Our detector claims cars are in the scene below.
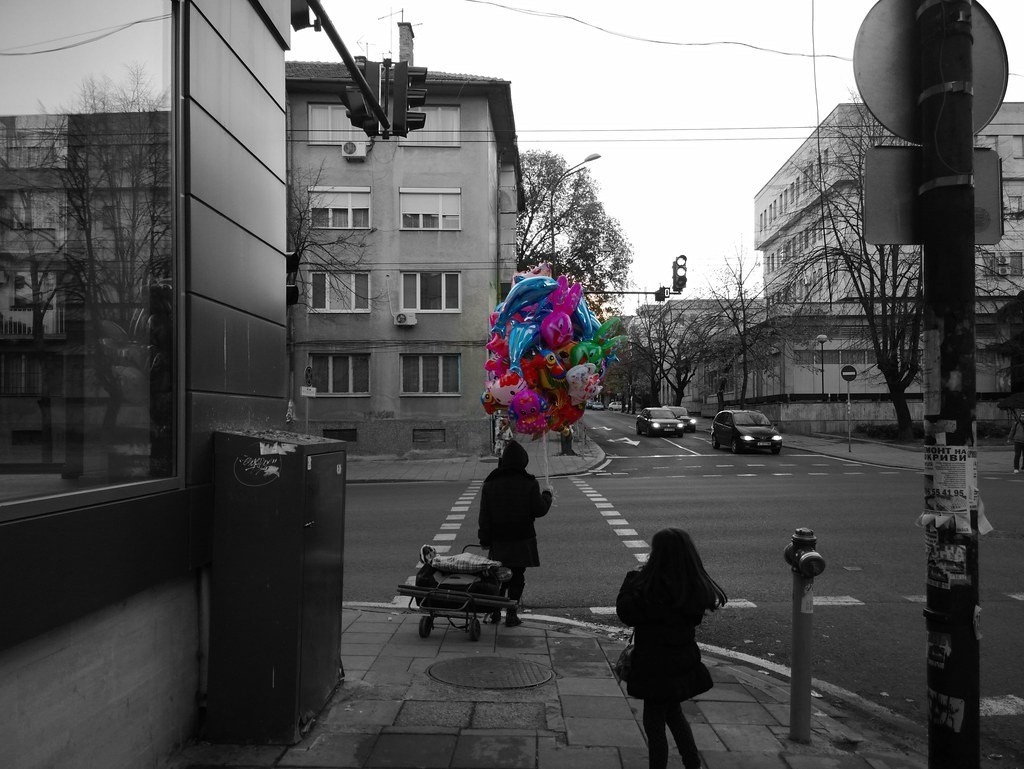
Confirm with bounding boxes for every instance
[586,401,605,410]
[664,406,696,432]
[711,410,783,454]
[608,402,629,411]
[637,407,685,439]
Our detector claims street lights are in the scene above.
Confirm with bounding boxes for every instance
[817,335,828,396]
[549,153,602,280]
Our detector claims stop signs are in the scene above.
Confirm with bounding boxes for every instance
[841,365,857,381]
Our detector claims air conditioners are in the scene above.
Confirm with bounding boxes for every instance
[997,256,1010,265]
[394,312,415,326]
[342,142,366,159]
[998,267,1011,275]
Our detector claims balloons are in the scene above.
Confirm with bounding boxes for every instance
[481,261,630,439]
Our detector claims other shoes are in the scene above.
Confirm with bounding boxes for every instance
[1014,467,1024,473]
[491,611,502,624]
[506,612,523,627]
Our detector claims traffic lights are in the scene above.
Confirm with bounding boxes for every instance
[672,255,687,291]
[394,60,429,138]
[338,59,381,136]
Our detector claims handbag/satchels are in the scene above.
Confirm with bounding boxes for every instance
[615,645,634,681]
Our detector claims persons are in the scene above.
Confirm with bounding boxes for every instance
[478,441,554,627]
[1006,412,1024,473]
[616,527,728,769]
[494,419,513,469]
